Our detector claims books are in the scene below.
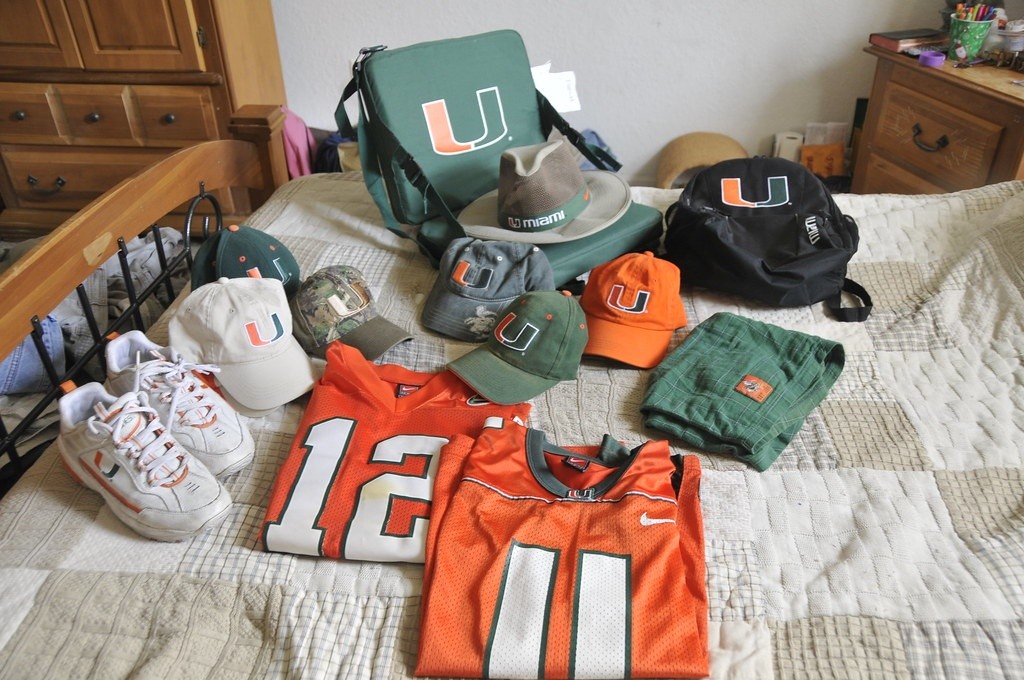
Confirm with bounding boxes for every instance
[869,27,951,54]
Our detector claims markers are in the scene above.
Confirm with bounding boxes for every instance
[956,2,997,21]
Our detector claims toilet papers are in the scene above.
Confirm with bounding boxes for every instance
[773,131,804,169]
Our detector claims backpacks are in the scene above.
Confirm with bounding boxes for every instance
[663,154,874,320]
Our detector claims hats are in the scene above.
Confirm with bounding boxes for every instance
[418,135,688,409]
[166,224,413,413]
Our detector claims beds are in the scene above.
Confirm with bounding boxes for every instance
[0,102,1024,679]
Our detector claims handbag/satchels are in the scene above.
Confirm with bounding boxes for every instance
[352,29,550,227]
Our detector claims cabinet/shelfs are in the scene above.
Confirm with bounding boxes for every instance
[846,31,1024,193]
[0,1,293,242]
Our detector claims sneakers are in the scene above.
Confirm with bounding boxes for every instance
[54,330,255,541]
